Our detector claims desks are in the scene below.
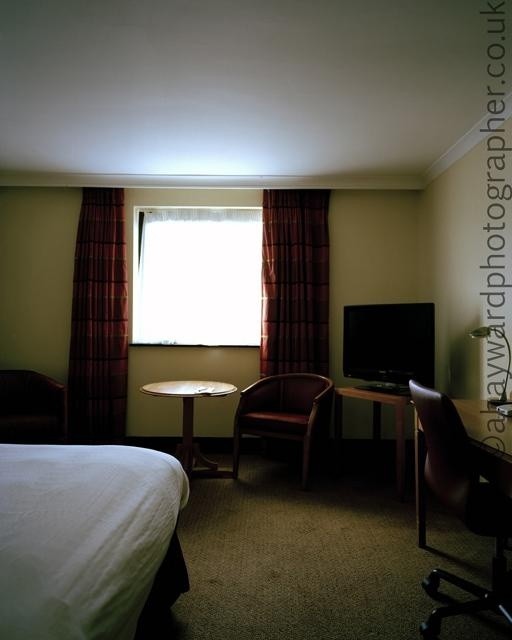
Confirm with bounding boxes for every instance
[333,386,411,506]
[410,399,512,547]
[138,379,238,478]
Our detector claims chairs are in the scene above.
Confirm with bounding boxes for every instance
[232,372,335,492]
[0,371,68,445]
[408,379,512,640]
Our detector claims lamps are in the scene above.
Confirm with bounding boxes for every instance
[469,327,512,406]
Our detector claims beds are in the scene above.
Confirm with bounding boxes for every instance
[1,445,189,640]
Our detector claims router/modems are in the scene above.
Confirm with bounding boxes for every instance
[496,403,512,416]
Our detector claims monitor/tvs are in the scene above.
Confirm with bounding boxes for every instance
[343,302,435,395]
